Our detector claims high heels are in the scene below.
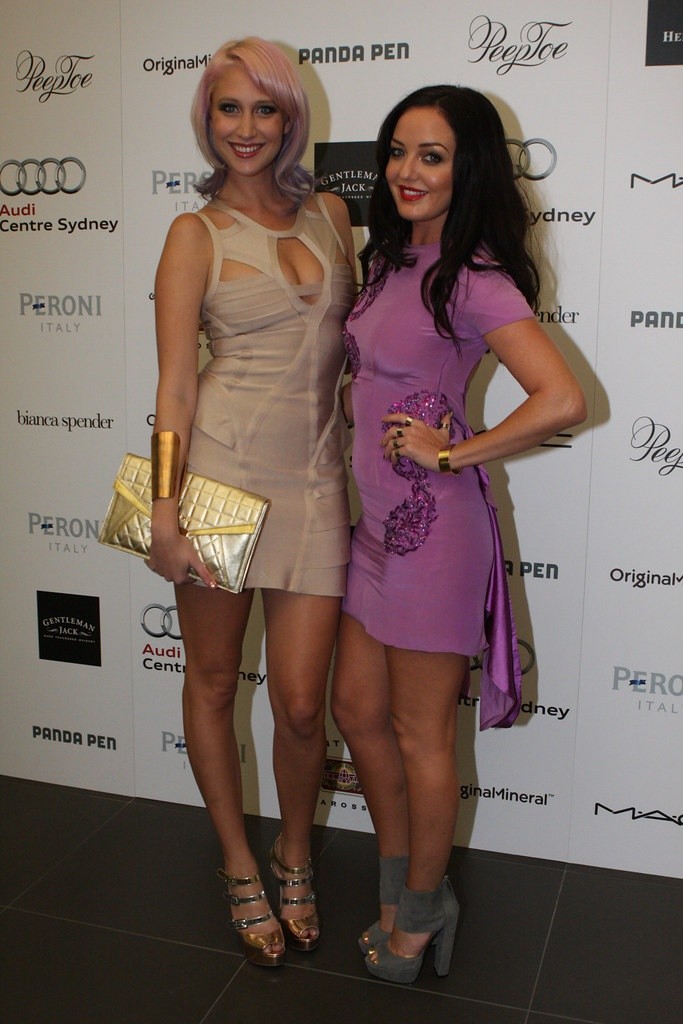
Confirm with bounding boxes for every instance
[268,838,320,952]
[366,875,461,982]
[218,859,286,968]
[356,855,416,956]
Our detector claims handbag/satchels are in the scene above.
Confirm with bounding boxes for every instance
[98,452,270,595]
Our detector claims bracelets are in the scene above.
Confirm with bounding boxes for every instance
[438,444,463,477]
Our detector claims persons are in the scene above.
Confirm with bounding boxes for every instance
[149,37,357,968]
[329,84,587,984]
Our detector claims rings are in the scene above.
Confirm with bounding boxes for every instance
[395,448,400,457]
[439,423,450,430]
[392,437,399,448]
[405,415,413,426]
[397,427,403,437]
